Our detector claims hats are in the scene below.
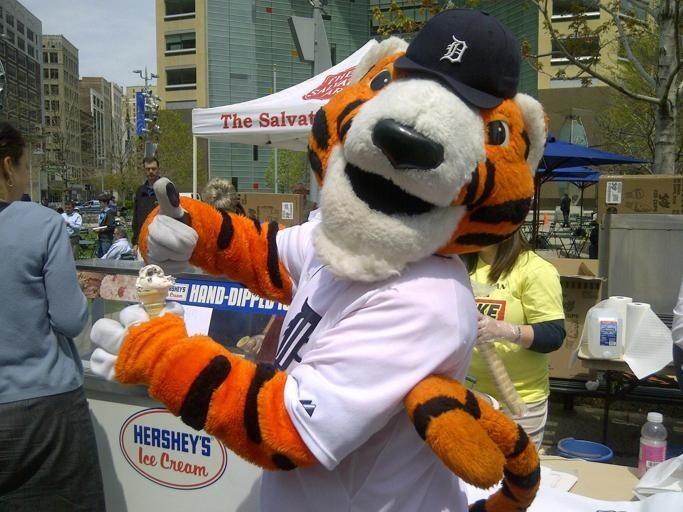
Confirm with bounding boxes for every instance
[98,208,125,226]
[74,200,102,214]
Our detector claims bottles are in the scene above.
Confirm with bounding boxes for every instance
[393,9,521,109]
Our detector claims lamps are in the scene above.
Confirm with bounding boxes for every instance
[121,251,135,260]
[79,230,95,258]
[523,216,592,259]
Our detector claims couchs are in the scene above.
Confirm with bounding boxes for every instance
[84,184,88,191]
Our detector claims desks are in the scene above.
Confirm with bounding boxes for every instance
[132,66,163,158]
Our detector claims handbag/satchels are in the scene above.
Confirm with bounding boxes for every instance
[512,325,523,341]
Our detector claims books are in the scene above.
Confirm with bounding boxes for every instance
[547,260,606,382]
[239,193,304,228]
[597,176,683,214]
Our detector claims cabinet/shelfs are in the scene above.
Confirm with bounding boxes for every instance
[637,411,668,482]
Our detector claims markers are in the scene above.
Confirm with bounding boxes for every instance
[135,265,177,319]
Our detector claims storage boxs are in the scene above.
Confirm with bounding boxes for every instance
[543,314,682,445]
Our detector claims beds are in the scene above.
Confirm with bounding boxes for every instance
[528,133,655,258]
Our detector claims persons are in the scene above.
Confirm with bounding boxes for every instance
[669,281,683,389]
[463,229,568,455]
[201,177,244,217]
[0,124,106,509]
[92,195,131,261]
[293,182,319,224]
[59,200,82,260]
[131,156,164,259]
